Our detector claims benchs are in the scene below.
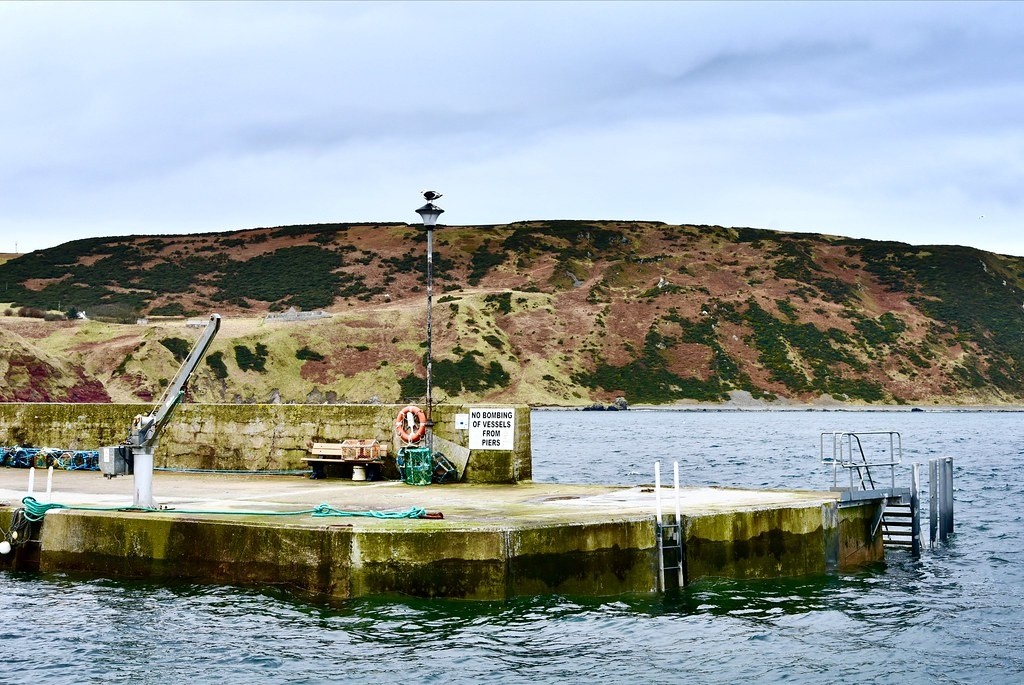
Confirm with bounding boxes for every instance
[186,320,210,328]
[300,442,387,480]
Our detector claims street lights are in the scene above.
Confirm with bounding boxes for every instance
[416,189,446,461]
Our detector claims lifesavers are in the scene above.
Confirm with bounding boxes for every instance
[396,406,426,442]
